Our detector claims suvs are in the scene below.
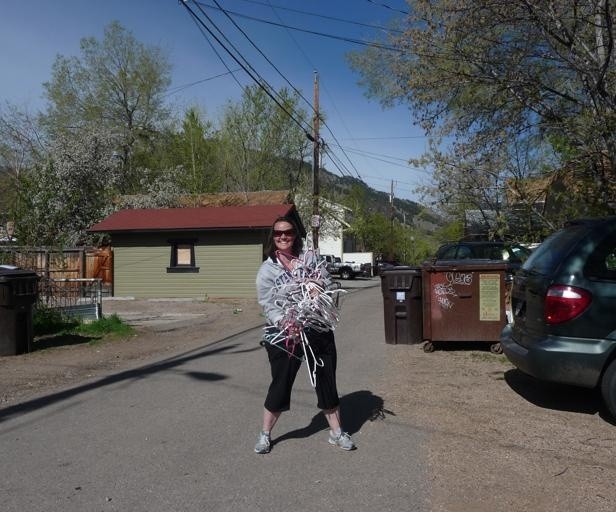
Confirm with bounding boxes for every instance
[500,215,616,418]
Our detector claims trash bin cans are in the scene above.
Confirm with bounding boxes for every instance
[0,265,41,357]
[377,259,511,354]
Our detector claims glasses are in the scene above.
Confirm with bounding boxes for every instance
[272,228,295,237]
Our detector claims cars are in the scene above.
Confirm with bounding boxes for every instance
[435,240,542,270]
[320,255,401,279]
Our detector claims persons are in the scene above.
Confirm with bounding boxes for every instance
[253,215,355,454]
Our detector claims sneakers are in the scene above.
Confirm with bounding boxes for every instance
[254,431,271,453]
[328,430,355,451]
